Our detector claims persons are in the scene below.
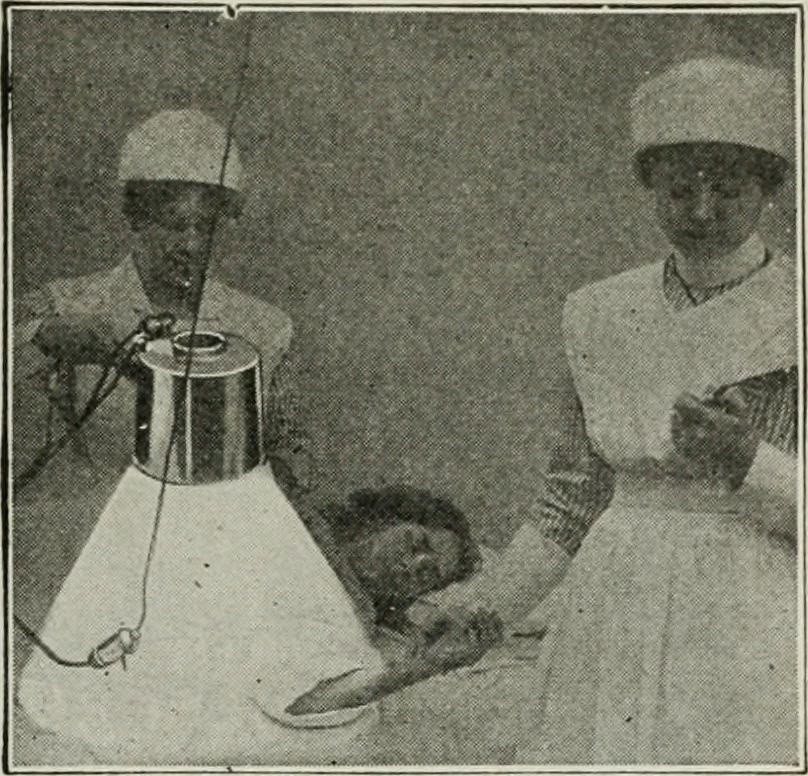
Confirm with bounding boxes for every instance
[12,108,293,678]
[286,483,505,718]
[398,53,797,766]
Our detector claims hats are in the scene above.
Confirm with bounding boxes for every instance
[627,54,795,190]
[118,108,242,195]
